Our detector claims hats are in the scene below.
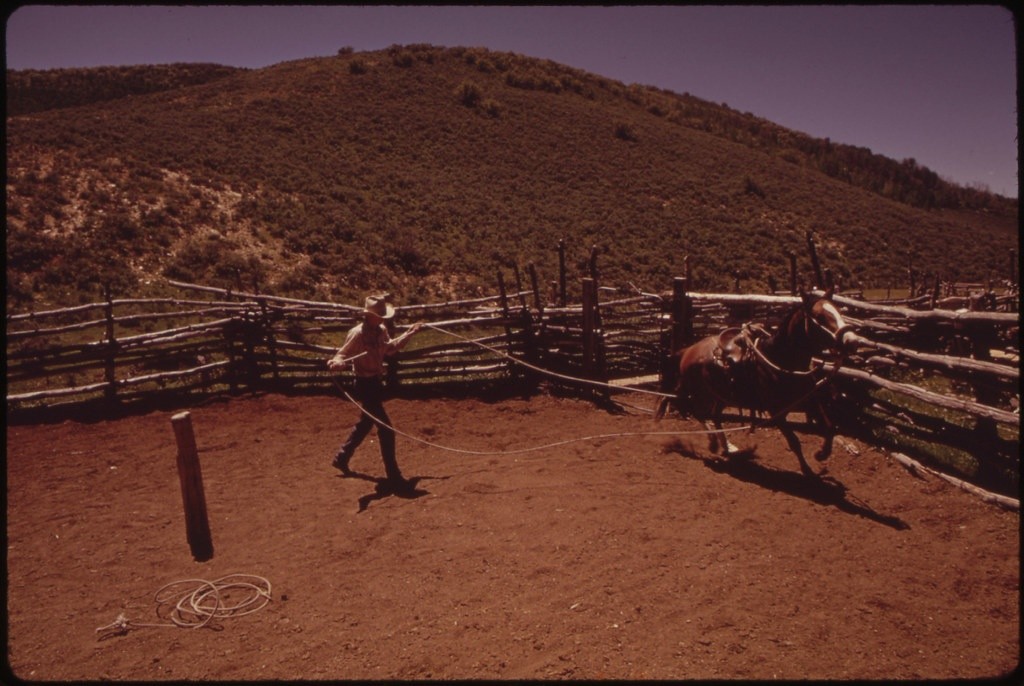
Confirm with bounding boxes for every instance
[358,295,395,319]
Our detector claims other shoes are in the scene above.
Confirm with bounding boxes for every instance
[389,477,410,487]
[332,459,357,476]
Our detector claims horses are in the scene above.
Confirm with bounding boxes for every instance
[655,284,858,486]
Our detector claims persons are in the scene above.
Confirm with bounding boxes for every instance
[326,294,422,492]
